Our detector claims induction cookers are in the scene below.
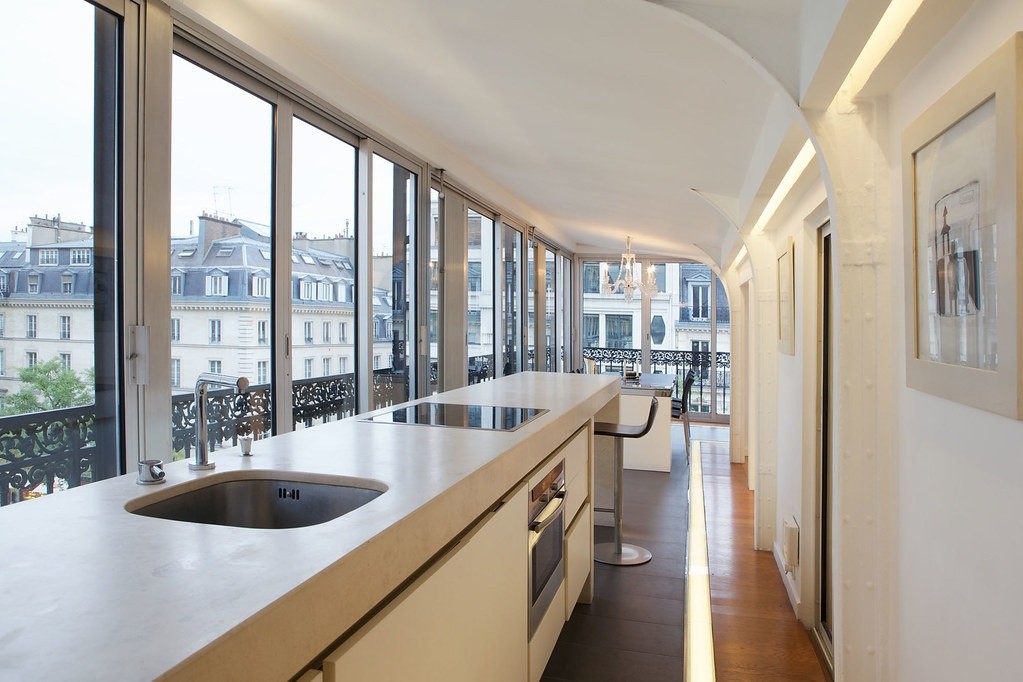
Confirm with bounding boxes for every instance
[355,401,550,433]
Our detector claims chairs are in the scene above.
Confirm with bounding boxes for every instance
[670,370,695,464]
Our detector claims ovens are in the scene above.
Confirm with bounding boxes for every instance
[527,459,568,642]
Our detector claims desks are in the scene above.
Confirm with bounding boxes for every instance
[601,371,677,397]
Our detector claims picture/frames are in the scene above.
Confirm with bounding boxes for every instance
[777,238,794,353]
[900,31,1023,423]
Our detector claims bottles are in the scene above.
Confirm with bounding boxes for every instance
[584,356,595,374]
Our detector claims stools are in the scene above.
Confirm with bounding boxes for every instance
[593,397,658,566]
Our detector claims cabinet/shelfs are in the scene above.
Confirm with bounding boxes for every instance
[297,418,595,682]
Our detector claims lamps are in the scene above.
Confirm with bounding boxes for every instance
[600,236,658,304]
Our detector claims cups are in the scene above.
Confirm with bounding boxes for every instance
[239,437,252,455]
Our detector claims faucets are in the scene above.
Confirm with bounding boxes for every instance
[188,372,249,471]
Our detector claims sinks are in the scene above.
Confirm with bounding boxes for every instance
[130,480,384,529]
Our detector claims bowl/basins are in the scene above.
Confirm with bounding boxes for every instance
[619,371,642,379]
